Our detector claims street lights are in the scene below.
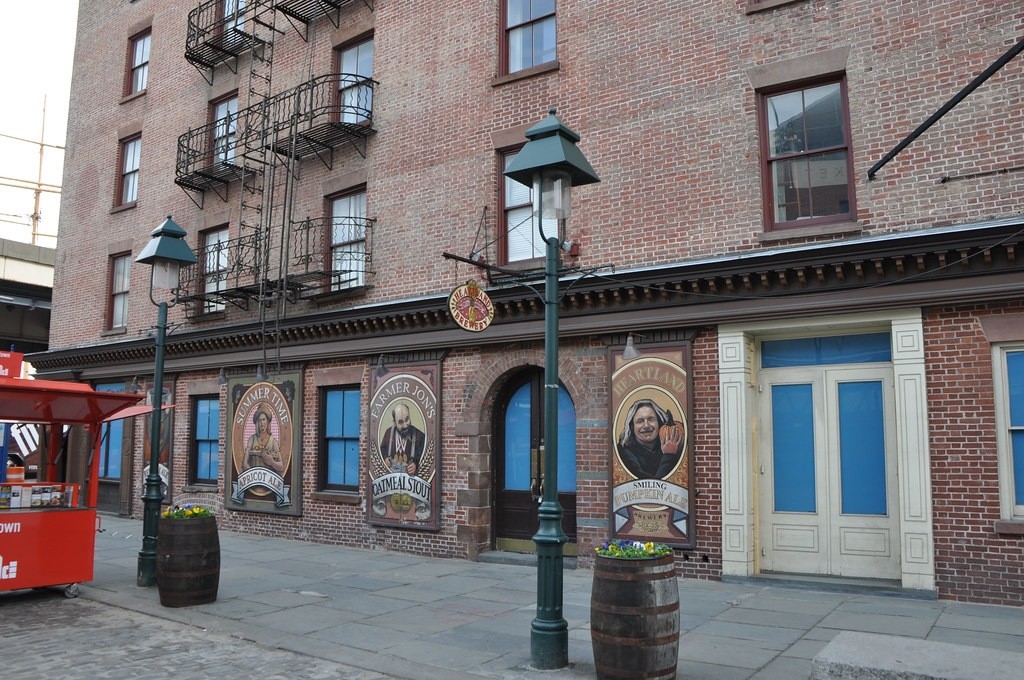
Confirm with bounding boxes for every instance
[501,108,601,665]
[133,213,199,587]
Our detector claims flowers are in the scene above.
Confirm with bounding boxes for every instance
[592,539,673,558]
[160,504,214,519]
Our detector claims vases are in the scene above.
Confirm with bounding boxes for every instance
[590,554,681,680]
[157,516,220,608]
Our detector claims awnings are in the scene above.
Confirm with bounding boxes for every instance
[0,293,51,310]
[0,382,176,466]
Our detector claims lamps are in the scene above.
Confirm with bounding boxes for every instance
[562,240,579,257]
[623,332,648,361]
[256,365,284,382]
[373,354,404,375]
[471,251,486,264]
[217,366,246,385]
[130,376,154,392]
